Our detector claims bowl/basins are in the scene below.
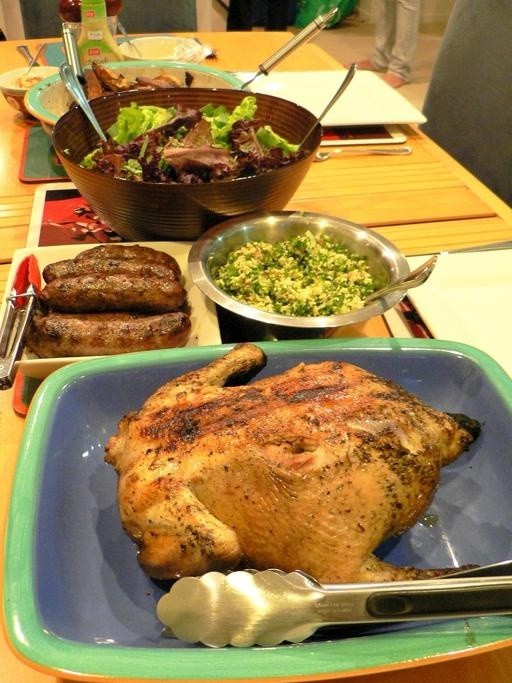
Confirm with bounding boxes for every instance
[188,211,412,327]
[0,66,64,126]
[23,60,254,141]
[118,36,206,63]
[50,87,324,243]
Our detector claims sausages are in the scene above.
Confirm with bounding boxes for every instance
[29,245,192,360]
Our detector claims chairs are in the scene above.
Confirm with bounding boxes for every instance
[18,0,198,40]
[417,0,512,207]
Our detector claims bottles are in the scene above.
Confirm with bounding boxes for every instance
[75,1,124,75]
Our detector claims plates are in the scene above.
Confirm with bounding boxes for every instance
[2,242,224,378]
[234,68,428,127]
[0,339,510,682]
[381,248,510,375]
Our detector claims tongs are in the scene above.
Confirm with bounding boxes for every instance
[0,254,38,391]
[156,558,511,648]
